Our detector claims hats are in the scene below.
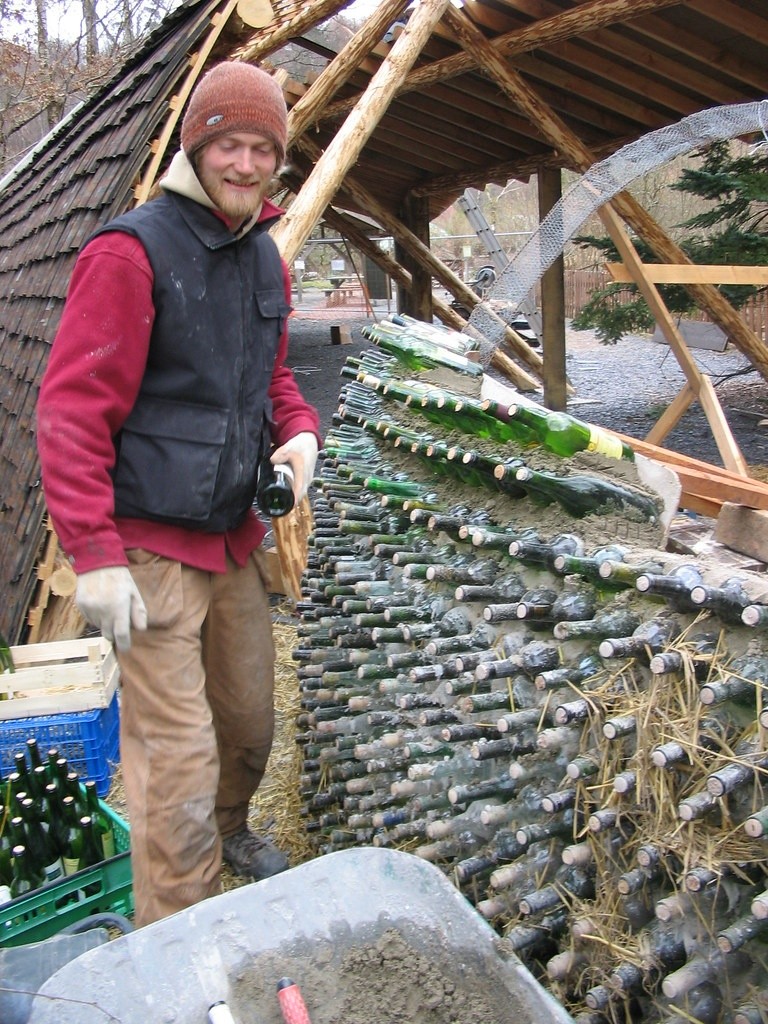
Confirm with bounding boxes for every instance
[181,61,287,176]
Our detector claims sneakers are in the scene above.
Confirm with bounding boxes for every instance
[222,826,288,879]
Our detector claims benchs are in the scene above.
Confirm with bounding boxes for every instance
[321,283,364,303]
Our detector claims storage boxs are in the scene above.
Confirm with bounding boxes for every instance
[0,691,136,949]
[331,324,354,346]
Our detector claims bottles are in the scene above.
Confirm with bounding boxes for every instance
[0,739,115,901]
[0,633,17,701]
[259,312,768,1024]
[276,977,311,1024]
[208,1000,235,1024]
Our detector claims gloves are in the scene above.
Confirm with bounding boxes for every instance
[75,564,149,652]
[269,430,318,509]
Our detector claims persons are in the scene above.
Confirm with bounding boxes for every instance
[36,59,320,931]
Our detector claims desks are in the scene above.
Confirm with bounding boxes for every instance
[326,276,365,301]
